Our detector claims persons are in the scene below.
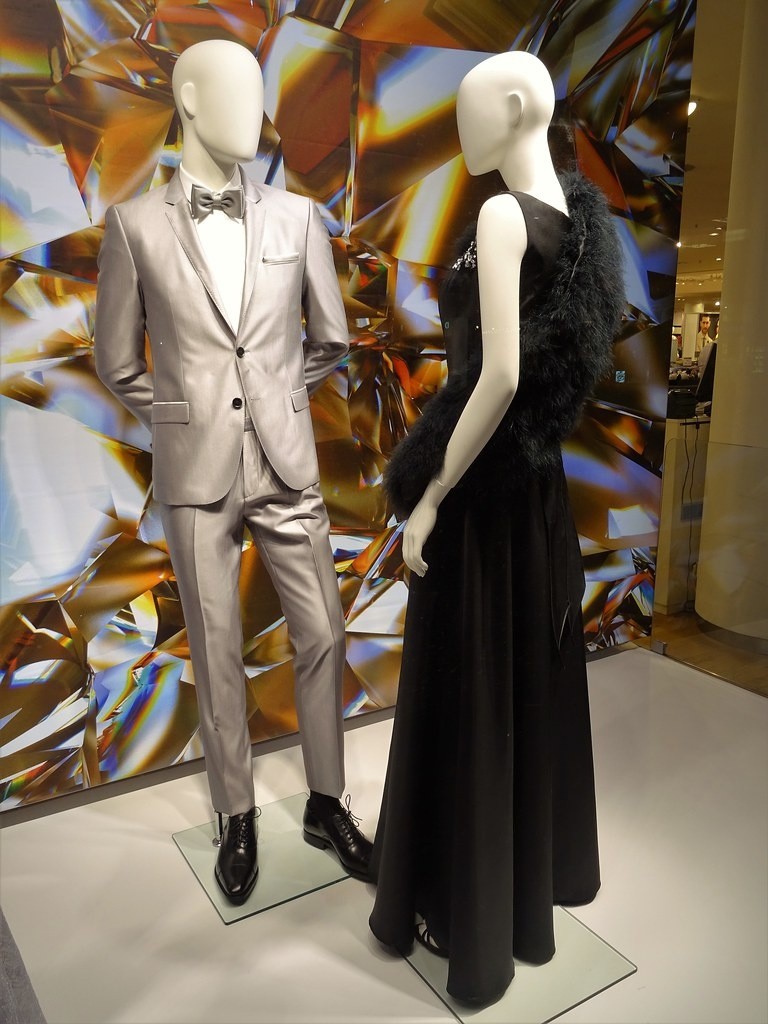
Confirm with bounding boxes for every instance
[697,314,713,352]
[93,39,371,904]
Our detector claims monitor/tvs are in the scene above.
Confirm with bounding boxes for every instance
[695,343,717,404]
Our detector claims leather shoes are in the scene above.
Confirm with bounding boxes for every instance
[303,797,377,885]
[214,807,258,906]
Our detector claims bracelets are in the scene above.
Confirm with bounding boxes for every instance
[369,51,625,1007]
[431,475,451,495]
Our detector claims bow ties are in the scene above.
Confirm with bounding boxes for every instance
[191,184,247,223]
